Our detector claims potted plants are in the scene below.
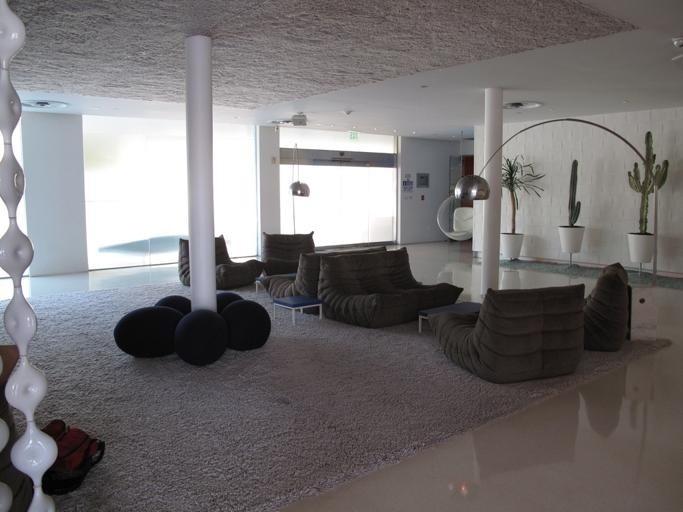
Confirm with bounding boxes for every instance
[626,131,668,263]
[500,154,545,259]
[556,160,586,253]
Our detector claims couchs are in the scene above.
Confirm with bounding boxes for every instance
[260,245,387,300]
[316,246,465,329]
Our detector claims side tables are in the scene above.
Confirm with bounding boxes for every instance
[418,301,482,334]
[273,294,322,327]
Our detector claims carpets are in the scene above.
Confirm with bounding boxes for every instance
[0,274,672,511]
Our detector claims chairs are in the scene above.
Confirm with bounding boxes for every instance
[583,261,627,352]
[0,344,35,511]
[178,234,265,290]
[437,194,473,241]
[261,231,314,274]
[426,282,585,384]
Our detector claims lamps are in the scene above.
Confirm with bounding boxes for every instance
[454,117,657,289]
[289,143,310,235]
